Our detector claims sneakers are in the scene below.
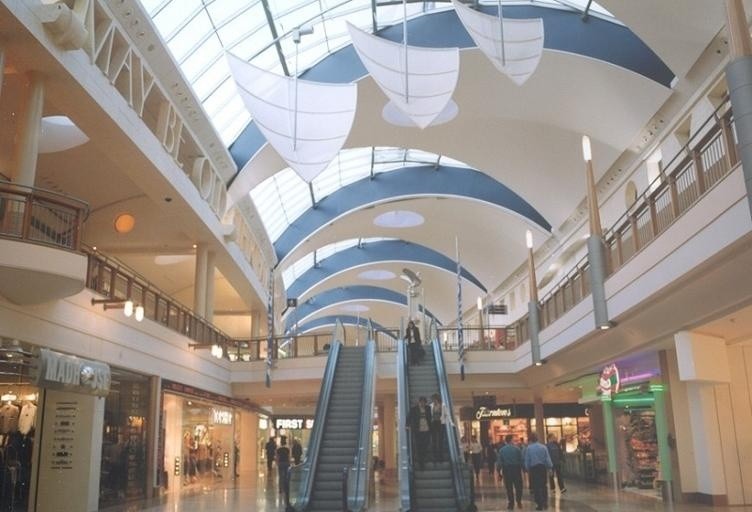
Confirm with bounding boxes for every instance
[507,497,523,510]
[551,488,567,495]
[535,502,548,511]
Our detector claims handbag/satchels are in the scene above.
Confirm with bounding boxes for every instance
[418,345,426,359]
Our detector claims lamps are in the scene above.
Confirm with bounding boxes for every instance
[91,295,147,322]
[191,339,225,358]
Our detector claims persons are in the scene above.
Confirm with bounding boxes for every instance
[264,437,303,493]
[404,321,422,368]
[109,428,147,498]
[186,433,200,482]
[461,430,568,510]
[5,430,27,505]
[496,342,506,351]
[407,392,456,471]
